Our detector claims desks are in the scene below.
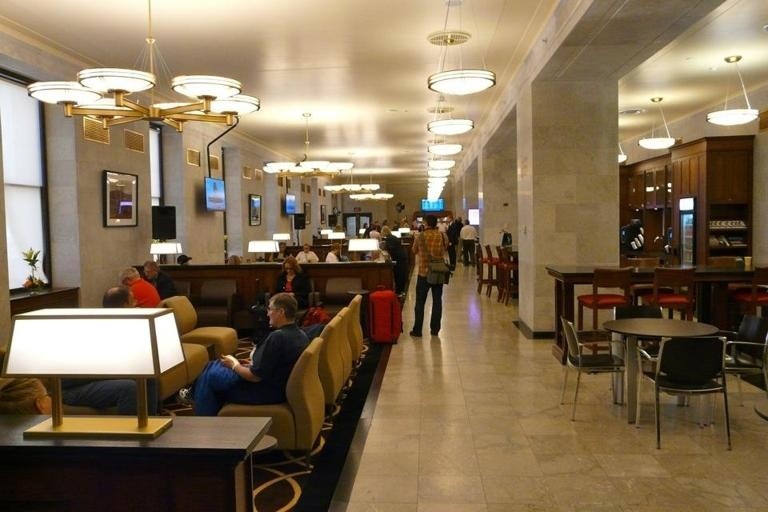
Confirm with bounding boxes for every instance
[0,412,276,512]
[600,317,720,424]
[543,260,766,370]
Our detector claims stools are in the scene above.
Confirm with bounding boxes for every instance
[575,266,632,372]
[642,265,698,322]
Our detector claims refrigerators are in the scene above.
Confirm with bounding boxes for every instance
[679,197,695,265]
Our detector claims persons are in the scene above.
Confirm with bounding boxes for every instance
[365,226,409,294]
[1,377,53,417]
[228,255,241,265]
[326,243,349,262]
[120,267,161,308]
[447,216,464,263]
[296,243,319,263]
[103,285,138,308]
[410,215,450,338]
[249,256,313,346]
[459,219,479,268]
[176,293,309,417]
[177,255,192,265]
[363,213,410,242]
[60,378,157,416]
[269,241,294,262]
[143,261,177,300]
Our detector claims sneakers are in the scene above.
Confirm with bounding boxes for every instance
[410,331,422,336]
[176,387,192,407]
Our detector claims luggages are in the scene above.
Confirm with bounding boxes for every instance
[368,285,403,344]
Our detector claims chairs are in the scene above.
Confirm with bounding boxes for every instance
[632,333,735,452]
[192,278,240,329]
[315,276,362,318]
[263,272,313,321]
[558,315,626,422]
[732,266,766,321]
[715,311,766,414]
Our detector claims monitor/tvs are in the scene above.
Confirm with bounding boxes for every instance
[285,193,296,213]
[204,176,226,212]
[421,198,445,212]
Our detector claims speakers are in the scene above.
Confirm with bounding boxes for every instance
[294,214,305,229]
[151,205,174,241]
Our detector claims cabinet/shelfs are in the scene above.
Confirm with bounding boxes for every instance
[709,202,750,257]
[626,163,671,212]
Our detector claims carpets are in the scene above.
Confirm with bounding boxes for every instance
[154,334,386,512]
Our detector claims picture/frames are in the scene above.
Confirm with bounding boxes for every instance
[248,193,262,226]
[320,204,327,224]
[303,201,312,225]
[101,169,139,229]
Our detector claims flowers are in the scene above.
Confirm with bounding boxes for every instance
[21,247,45,291]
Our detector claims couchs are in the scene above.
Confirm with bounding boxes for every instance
[141,336,211,401]
[156,291,239,358]
[216,334,328,452]
[318,307,356,418]
[346,294,366,371]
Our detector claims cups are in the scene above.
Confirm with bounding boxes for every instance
[744,257,752,265]
[744,265,752,272]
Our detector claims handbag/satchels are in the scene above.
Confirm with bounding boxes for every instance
[427,261,450,284]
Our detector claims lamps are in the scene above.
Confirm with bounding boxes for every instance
[704,55,761,127]
[261,112,355,177]
[425,1,499,179]
[0,305,188,440]
[618,141,628,165]
[24,0,262,134]
[637,96,677,151]
[149,230,380,265]
[322,167,381,193]
